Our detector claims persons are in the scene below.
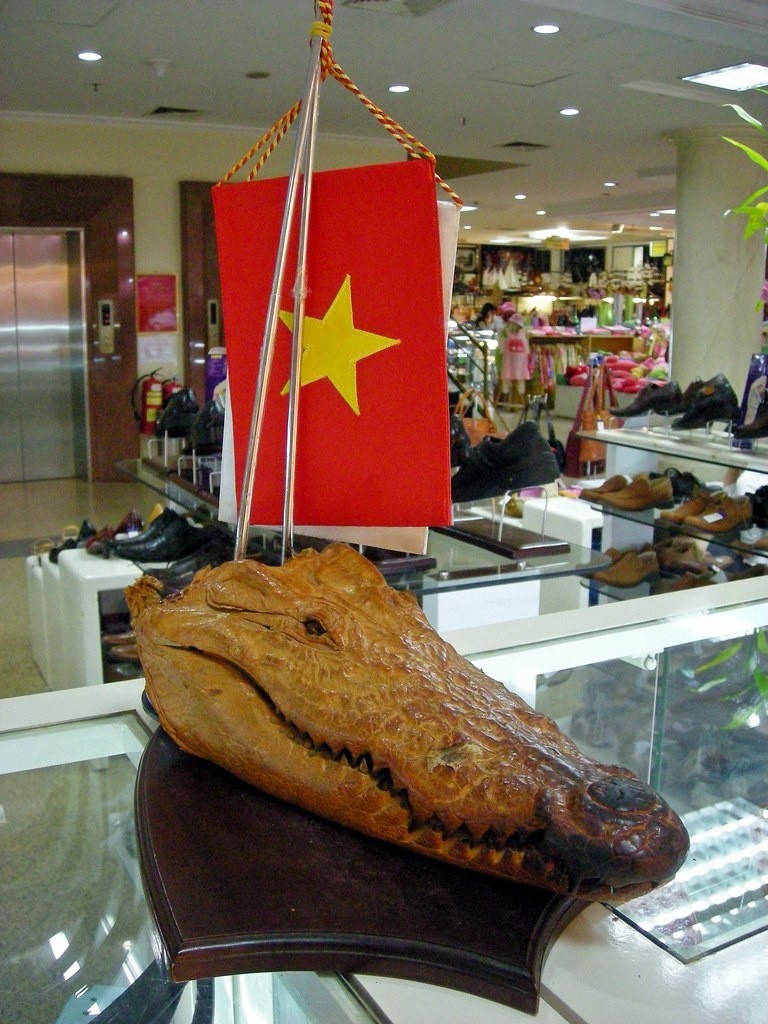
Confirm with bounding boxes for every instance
[498,313,531,410]
[496,302,516,329]
[476,304,495,328]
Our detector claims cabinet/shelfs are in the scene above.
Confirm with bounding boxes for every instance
[577,430,768,601]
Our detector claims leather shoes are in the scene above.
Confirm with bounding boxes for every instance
[450,420,560,503]
[608,371,767,442]
[577,464,768,598]
[35,385,341,665]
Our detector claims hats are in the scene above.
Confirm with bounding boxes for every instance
[509,313,525,326]
[498,301,516,314]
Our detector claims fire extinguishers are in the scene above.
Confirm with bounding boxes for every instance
[131,366,182,436]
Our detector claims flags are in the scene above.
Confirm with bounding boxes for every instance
[218,198,457,553]
[213,158,453,529]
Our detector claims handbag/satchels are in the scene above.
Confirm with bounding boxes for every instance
[452,387,566,471]
[560,364,624,478]
